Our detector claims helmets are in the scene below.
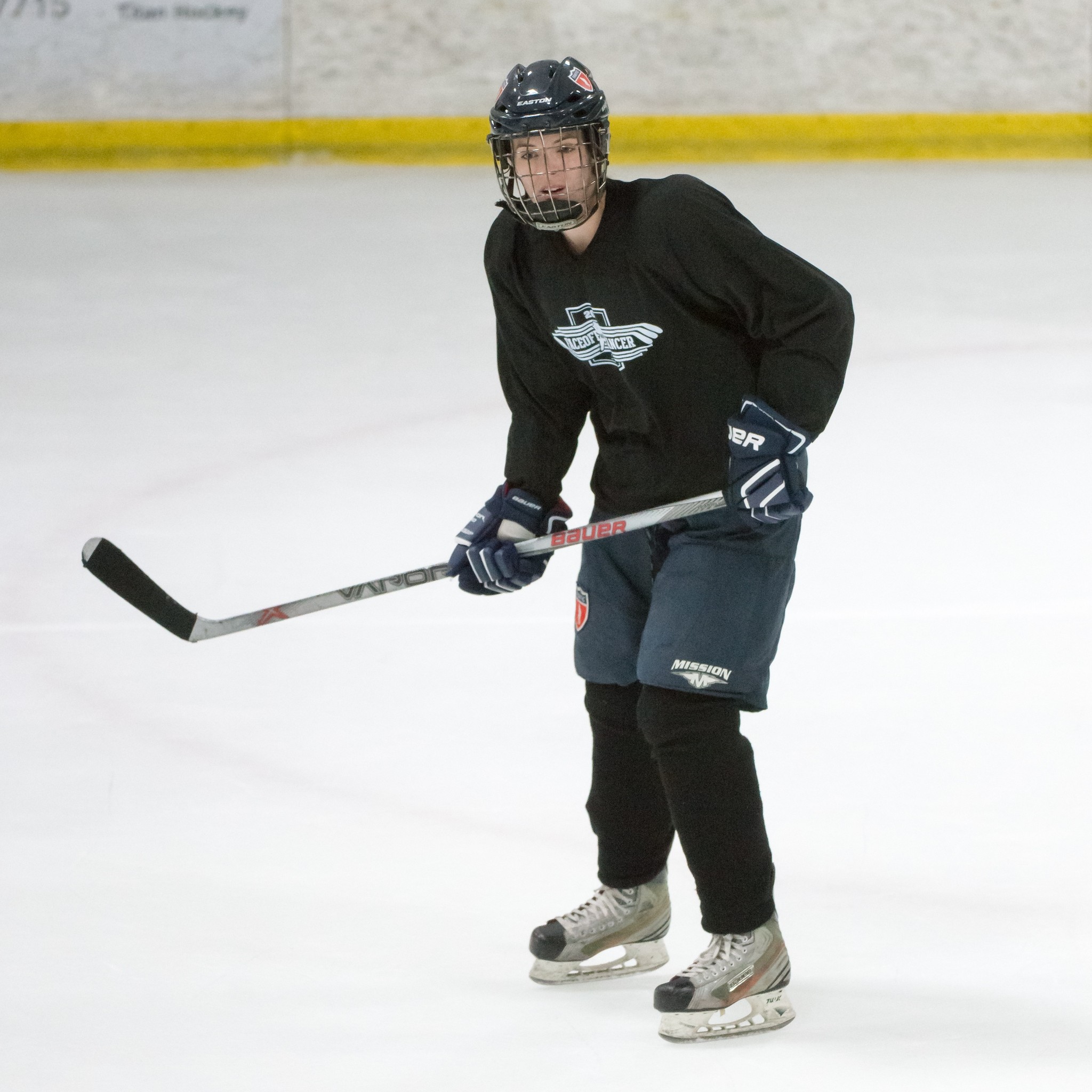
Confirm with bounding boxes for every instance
[485,58,609,233]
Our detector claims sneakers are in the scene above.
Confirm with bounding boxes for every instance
[655,864,796,1039]
[528,861,672,982]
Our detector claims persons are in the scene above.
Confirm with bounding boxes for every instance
[446,56,855,1039]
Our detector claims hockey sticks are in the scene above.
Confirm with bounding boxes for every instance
[81,487,729,643]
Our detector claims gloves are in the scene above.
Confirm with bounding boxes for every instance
[721,395,813,525]
[446,478,573,595]
